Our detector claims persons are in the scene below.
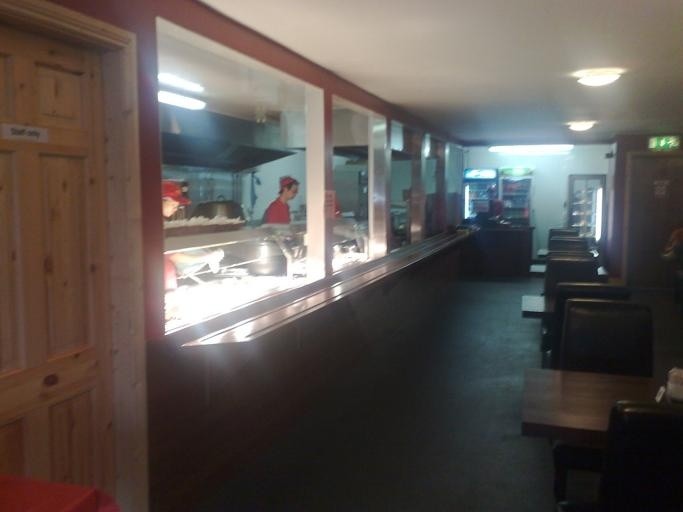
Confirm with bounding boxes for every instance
[160,180,191,295]
[261,176,299,223]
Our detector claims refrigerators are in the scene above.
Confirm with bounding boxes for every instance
[464,166,534,226]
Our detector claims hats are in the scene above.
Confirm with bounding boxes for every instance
[161,181,192,205]
[281,179,296,186]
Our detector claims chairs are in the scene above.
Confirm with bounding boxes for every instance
[545,228,681,511]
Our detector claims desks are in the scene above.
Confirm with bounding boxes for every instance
[521,249,669,442]
